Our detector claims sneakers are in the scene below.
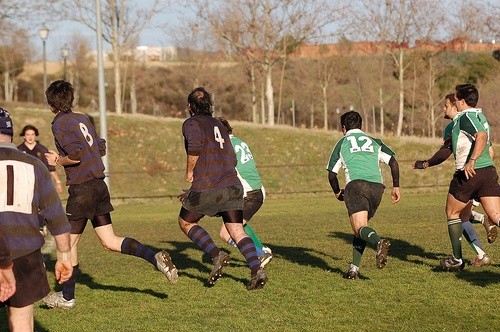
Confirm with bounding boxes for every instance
[41,291,75,310]
[246,269,269,291]
[470,254,491,267]
[439,255,465,272]
[482,212,497,243]
[375,238,391,269]
[206,250,231,288]
[154,249,179,285]
[258,246,274,269]
[346,269,359,279]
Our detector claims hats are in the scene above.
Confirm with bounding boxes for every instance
[0,108,14,132]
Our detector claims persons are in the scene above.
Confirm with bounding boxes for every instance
[212,117,273,268]
[44,81,179,309]
[326,111,399,280]
[178,87,268,289]
[0,108,73,332]
[16,125,62,192]
[413,83,500,271]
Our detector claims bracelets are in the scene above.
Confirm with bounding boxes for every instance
[55,156,62,167]
[470,158,475,160]
